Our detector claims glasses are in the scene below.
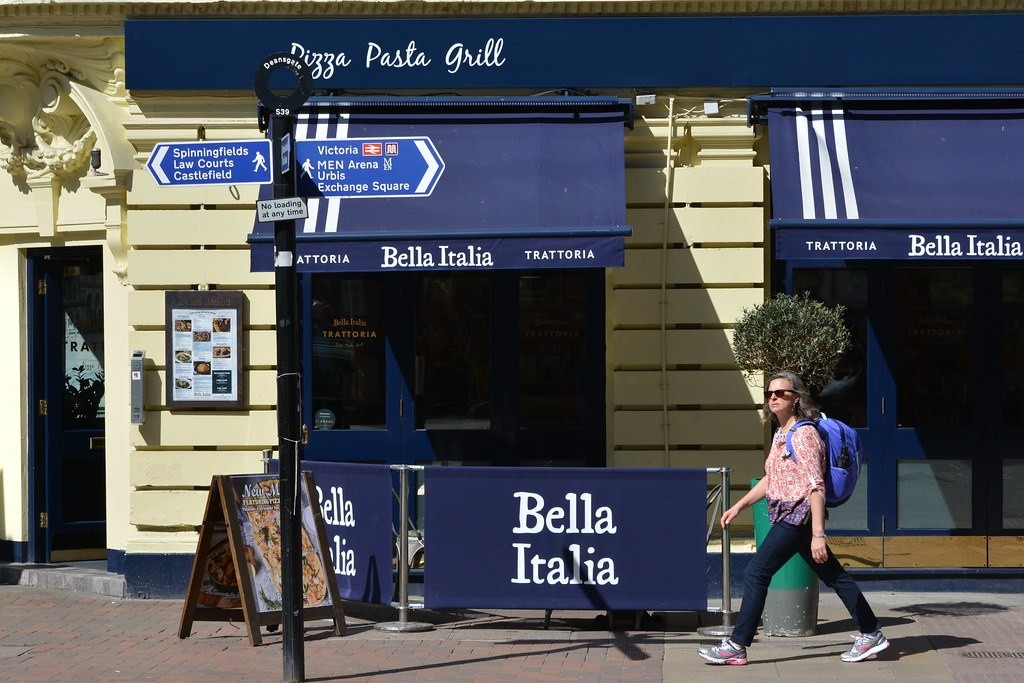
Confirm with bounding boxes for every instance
[763,389,797,398]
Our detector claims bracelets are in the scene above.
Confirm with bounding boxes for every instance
[812,533,827,538]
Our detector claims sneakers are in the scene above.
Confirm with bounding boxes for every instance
[841,631,890,663]
[696,639,747,665]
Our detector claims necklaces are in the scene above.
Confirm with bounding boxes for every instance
[779,417,795,431]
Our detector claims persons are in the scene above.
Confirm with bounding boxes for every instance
[697,370,890,666]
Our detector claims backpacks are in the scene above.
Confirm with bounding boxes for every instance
[786,411,862,507]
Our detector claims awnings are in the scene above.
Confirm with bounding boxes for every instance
[247,96,636,274]
[747,87,1024,261]
